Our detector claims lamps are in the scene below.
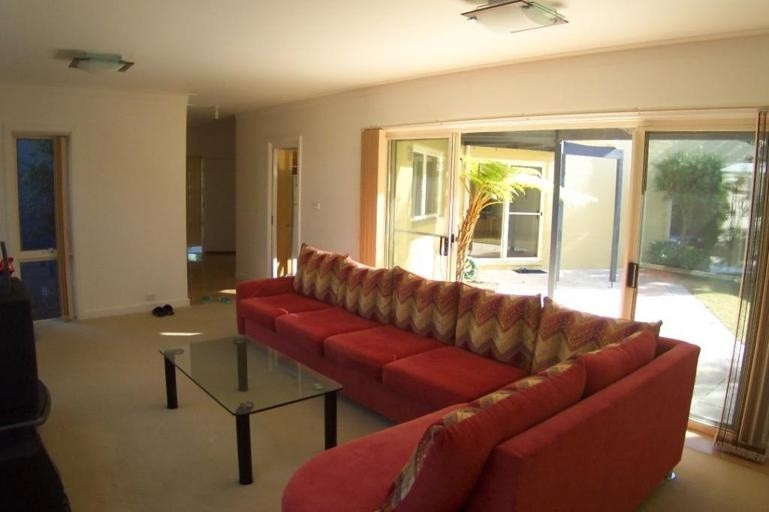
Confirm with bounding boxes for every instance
[64,52,134,77]
[460,1,568,37]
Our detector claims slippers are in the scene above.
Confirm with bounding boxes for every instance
[200,294,212,304]
[163,303,175,316]
[152,306,164,317]
[216,296,232,304]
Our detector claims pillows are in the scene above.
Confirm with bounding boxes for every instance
[295,244,394,326]
[390,265,457,345]
[455,280,542,372]
[577,320,662,399]
[529,295,650,376]
[378,358,587,512]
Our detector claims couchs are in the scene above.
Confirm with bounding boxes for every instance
[221,271,699,509]
